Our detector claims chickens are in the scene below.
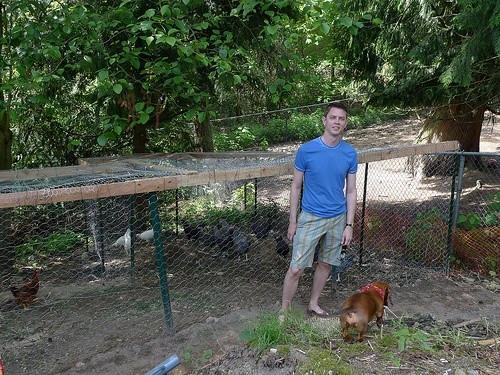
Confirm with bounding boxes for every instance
[110,217,290,258]
[11,270,40,310]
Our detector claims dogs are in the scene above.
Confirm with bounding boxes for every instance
[312,281,394,343]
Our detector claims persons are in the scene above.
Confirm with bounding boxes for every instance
[277,102,360,325]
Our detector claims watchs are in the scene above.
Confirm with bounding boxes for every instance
[345,223,356,228]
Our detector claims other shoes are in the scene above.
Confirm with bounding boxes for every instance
[306,308,328,317]
